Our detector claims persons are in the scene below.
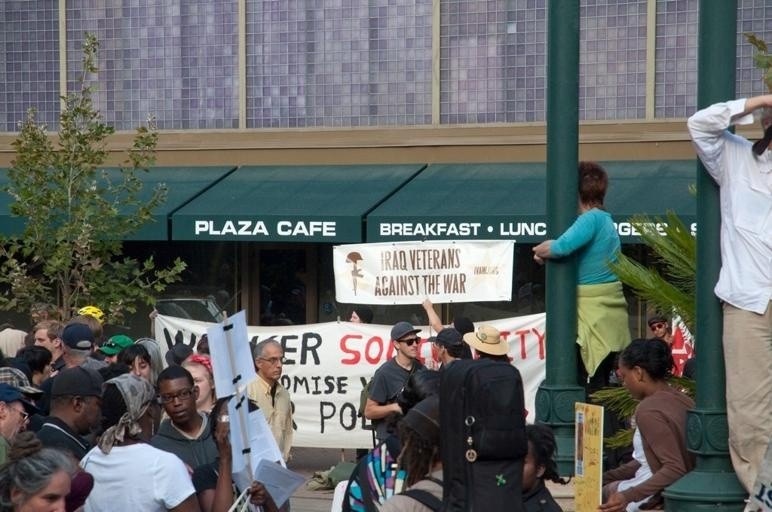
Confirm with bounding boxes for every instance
[686,93,772,512]
[532,160,632,469]
[270,258,307,324]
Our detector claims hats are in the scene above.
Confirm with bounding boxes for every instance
[402,393,441,447]
[390,321,423,340]
[433,329,463,348]
[0,382,41,419]
[97,334,134,356]
[0,366,45,401]
[50,363,111,404]
[462,324,510,356]
[648,316,667,327]
[59,322,95,351]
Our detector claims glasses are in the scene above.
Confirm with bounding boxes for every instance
[8,405,31,425]
[160,386,195,405]
[397,337,421,346]
[260,356,287,364]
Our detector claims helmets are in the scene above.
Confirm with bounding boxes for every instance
[77,306,105,328]
